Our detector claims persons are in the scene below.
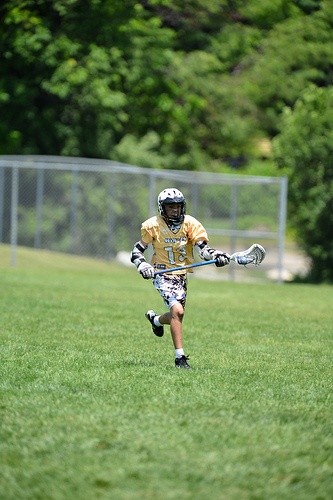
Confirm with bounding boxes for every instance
[131,188,230,369]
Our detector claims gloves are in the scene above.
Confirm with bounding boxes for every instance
[213,251,230,267]
[138,262,155,278]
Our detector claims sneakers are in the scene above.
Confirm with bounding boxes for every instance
[145,310,163,337]
[175,355,190,369]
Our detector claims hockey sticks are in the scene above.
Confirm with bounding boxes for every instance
[154,243,268,274]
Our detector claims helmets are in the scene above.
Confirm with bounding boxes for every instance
[158,188,185,216]
